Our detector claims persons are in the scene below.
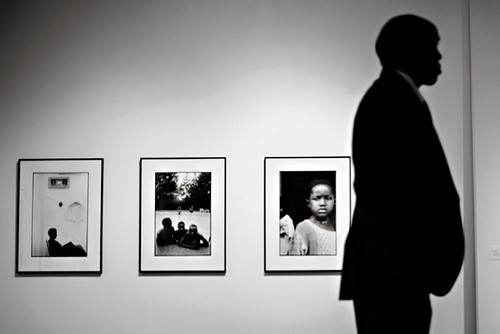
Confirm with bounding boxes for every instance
[339,10,466,334]
[156,218,181,248]
[179,224,209,251]
[174,221,189,245]
[47,228,86,257]
[292,180,336,255]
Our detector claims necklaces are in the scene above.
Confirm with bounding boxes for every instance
[310,215,330,226]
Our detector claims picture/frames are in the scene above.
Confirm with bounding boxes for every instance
[263,154,353,273]
[15,157,104,278]
[138,156,226,273]
